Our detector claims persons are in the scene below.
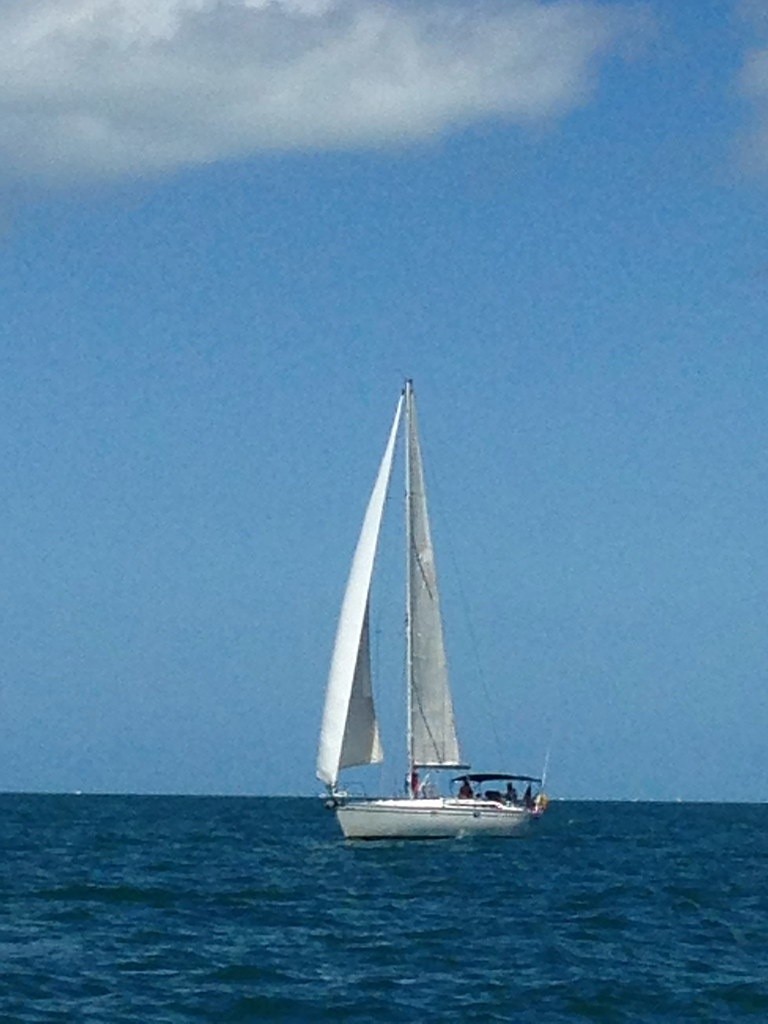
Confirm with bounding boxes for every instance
[503,781,517,804]
[458,781,472,799]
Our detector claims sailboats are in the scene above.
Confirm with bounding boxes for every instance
[315,378,549,835]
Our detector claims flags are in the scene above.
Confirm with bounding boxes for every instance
[404,772,419,794]
